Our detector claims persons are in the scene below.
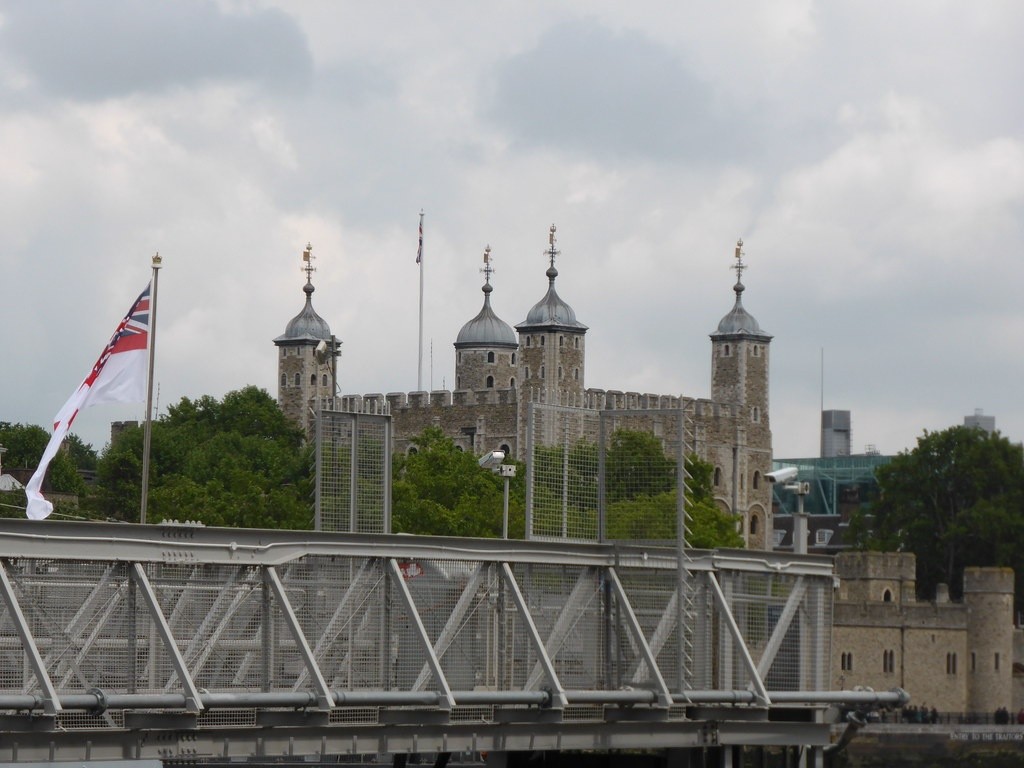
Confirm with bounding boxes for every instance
[902,705,929,723]
[881,710,886,721]
[930,708,938,723]
[995,707,1009,724]
[968,710,979,723]
[1017,708,1024,724]
[959,711,964,724]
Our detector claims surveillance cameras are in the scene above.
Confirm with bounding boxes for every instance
[479,450,505,469]
[763,467,799,483]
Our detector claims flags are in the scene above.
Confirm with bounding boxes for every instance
[24,277,151,521]
